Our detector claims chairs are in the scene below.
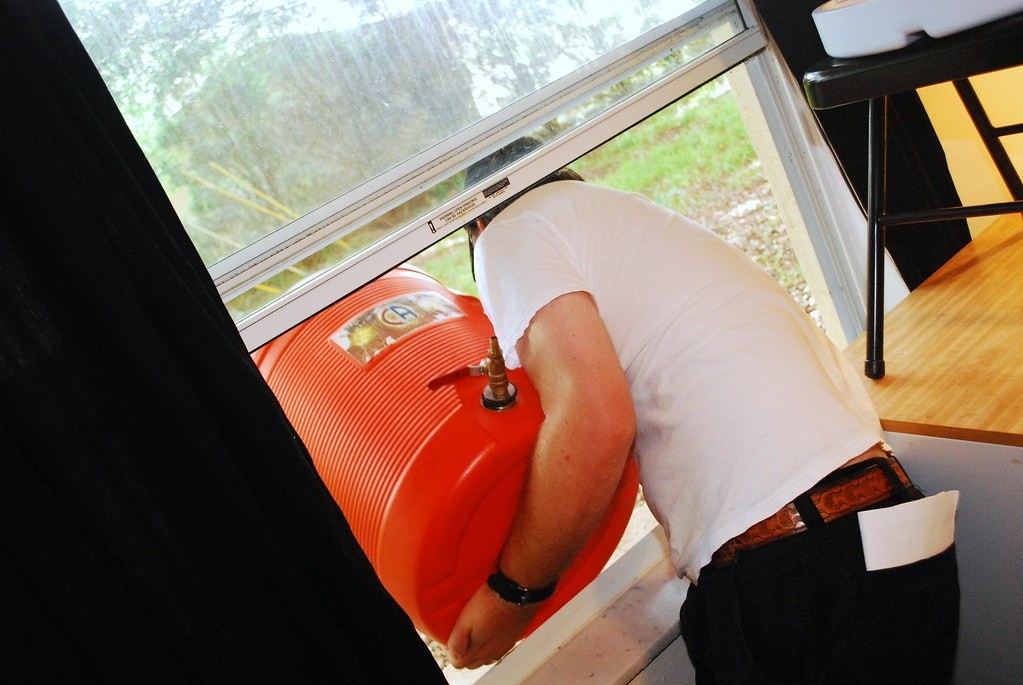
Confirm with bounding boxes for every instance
[756,0,1021,377]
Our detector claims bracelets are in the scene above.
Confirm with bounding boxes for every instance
[484,568,558,607]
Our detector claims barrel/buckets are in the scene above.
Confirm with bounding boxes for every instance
[243,260,642,658]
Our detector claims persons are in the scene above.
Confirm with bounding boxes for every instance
[439,135,959,685]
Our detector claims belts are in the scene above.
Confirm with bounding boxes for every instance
[709,457,913,564]
